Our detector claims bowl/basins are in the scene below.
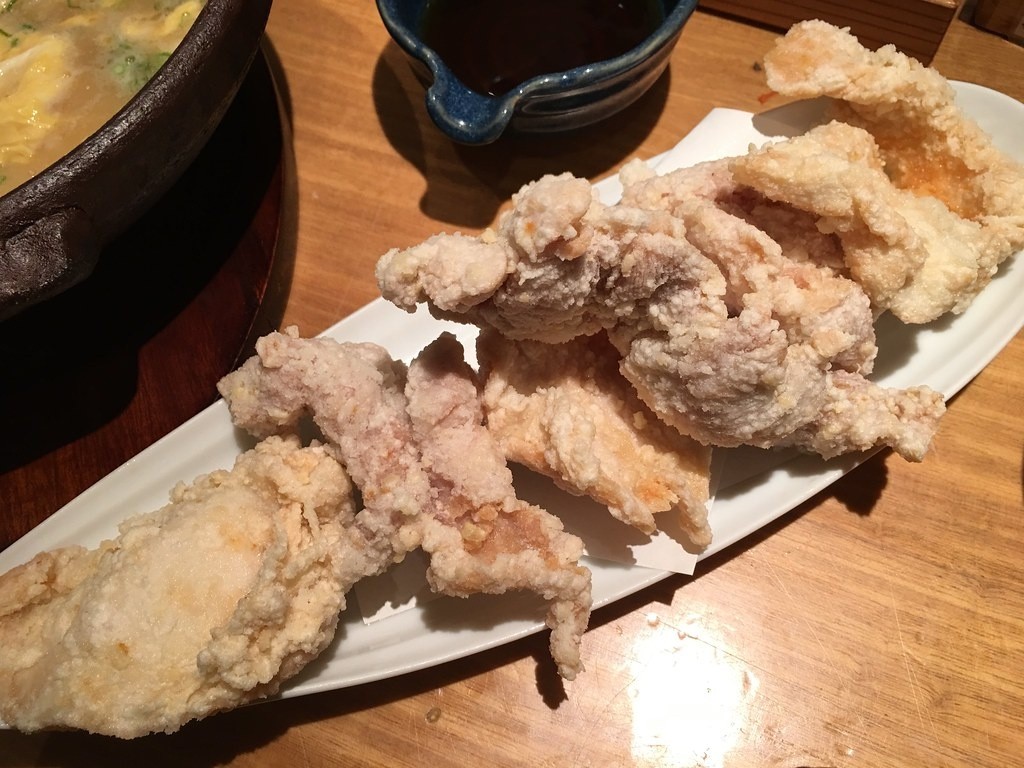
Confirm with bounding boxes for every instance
[367,0,703,150]
[1,2,275,311]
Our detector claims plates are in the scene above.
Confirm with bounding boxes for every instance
[1,71,1024,703]
